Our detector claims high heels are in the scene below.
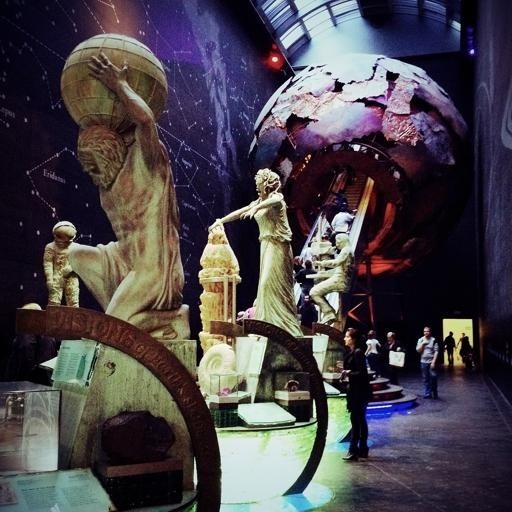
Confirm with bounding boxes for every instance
[342,449,358,461]
[358,448,369,458]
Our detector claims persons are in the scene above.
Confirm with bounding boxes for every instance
[444,332,456,365]
[68,48,190,341]
[309,234,353,326]
[416,326,444,401]
[364,328,384,378]
[205,168,302,337]
[457,331,473,361]
[293,251,318,324]
[338,327,372,462]
[383,329,408,385]
[42,221,84,310]
[314,192,357,248]
